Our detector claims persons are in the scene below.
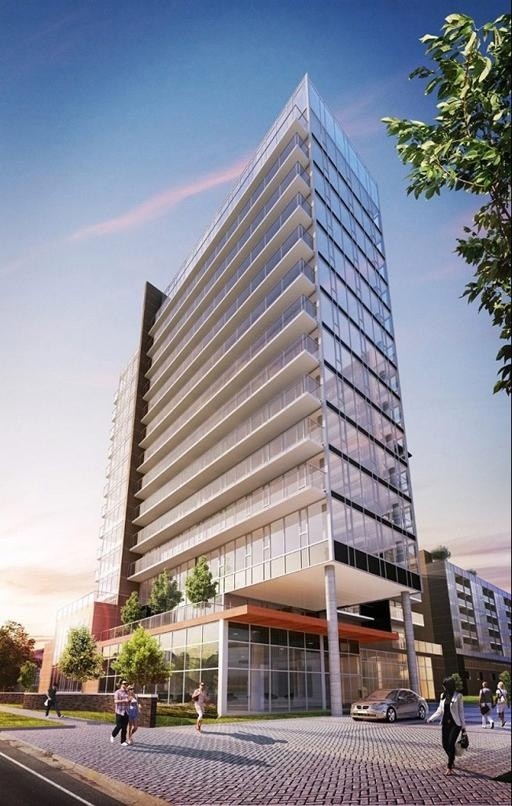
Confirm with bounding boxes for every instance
[479,681,495,729]
[493,681,508,727]
[126,685,139,746]
[192,682,209,735]
[110,679,130,745]
[45,681,63,719]
[425,676,466,775]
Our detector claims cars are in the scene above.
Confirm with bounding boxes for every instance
[350,689,430,721]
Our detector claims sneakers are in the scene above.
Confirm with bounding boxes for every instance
[110,734,134,746]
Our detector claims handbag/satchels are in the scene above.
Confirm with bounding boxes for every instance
[497,694,507,705]
[460,735,468,748]
[192,696,198,701]
[481,704,490,715]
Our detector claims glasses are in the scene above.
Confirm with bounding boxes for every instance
[129,688,134,690]
[121,683,128,685]
